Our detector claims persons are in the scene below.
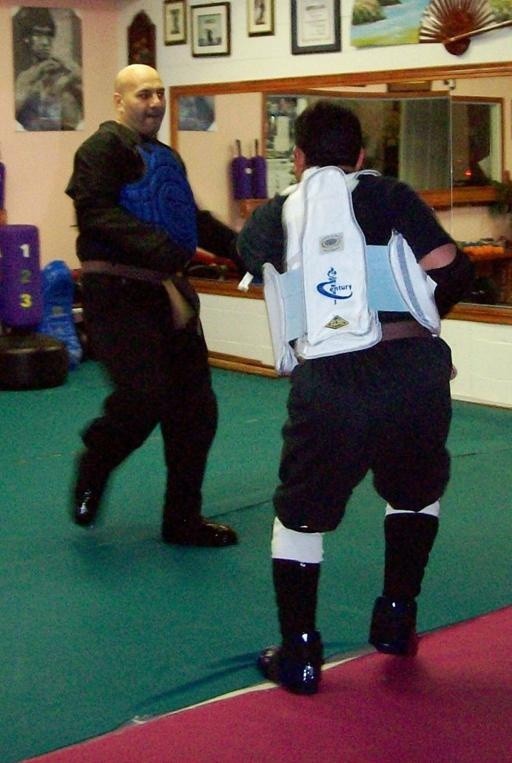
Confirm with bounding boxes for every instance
[376,108,402,178]
[60,59,256,548]
[13,5,85,130]
[236,94,478,695]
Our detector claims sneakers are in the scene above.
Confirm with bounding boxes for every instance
[256,632,324,696]
[73,453,108,527]
[368,595,420,657]
[162,516,238,547]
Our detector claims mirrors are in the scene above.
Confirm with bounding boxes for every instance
[264,90,505,210]
[169,61,512,326]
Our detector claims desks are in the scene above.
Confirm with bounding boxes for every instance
[463,245,512,307]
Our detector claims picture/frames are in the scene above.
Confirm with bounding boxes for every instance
[163,0,187,46]
[247,0,274,37]
[291,0,341,55]
[190,2,231,58]
